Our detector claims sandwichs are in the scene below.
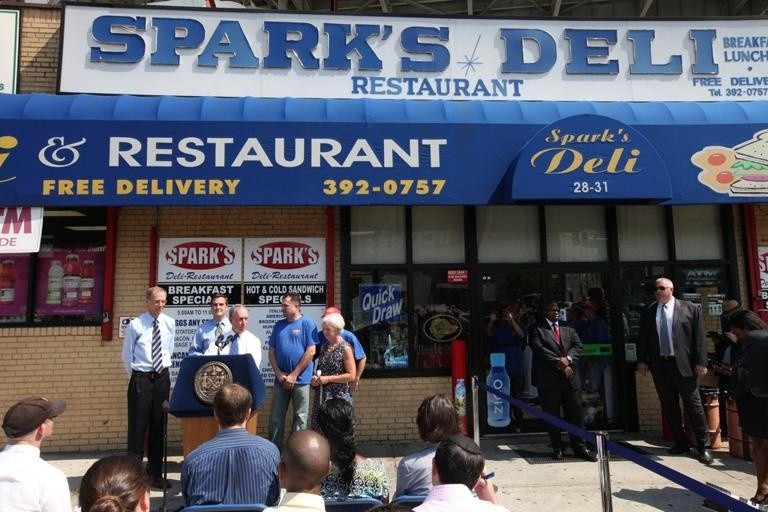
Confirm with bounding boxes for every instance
[728,128,768,196]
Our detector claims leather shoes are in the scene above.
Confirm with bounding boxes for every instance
[669,442,691,456]
[573,449,597,461]
[693,446,713,465]
[153,476,171,490]
[553,449,563,459]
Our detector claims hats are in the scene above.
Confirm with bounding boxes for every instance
[321,306,341,318]
[2,396,67,439]
[723,299,743,316]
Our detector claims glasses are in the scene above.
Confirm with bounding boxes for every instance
[654,285,670,291]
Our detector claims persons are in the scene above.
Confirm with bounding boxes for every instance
[712,300,744,441]
[311,313,352,432]
[254,430,333,512]
[392,393,459,501]
[203,304,261,368]
[268,292,321,453]
[318,307,367,392]
[484,304,530,434]
[528,301,597,462]
[120,286,175,489]
[79,456,150,512]
[188,293,231,357]
[637,278,713,464]
[366,504,415,512]
[182,384,282,507]
[405,435,503,512]
[0,396,71,512]
[316,398,389,504]
[731,310,768,504]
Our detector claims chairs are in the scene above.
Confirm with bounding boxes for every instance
[390,495,426,510]
[325,498,383,512]
[181,503,268,512]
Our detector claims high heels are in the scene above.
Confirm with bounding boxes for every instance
[750,491,768,504]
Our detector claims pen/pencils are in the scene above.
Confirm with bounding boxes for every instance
[481,472,496,481]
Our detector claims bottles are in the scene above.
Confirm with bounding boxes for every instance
[485,353,511,427]
[45,260,63,304]
[0,260,17,306]
[63,253,80,307]
[77,259,95,304]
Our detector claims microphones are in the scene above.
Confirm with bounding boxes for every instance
[222,335,233,348]
[215,332,224,348]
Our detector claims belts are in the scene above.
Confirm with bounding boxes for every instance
[132,370,167,380]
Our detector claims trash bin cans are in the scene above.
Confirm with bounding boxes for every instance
[723,389,754,462]
[699,385,721,449]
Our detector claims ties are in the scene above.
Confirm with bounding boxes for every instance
[553,323,561,343]
[660,306,672,357]
[152,320,165,373]
[216,323,227,340]
[229,334,241,355]
